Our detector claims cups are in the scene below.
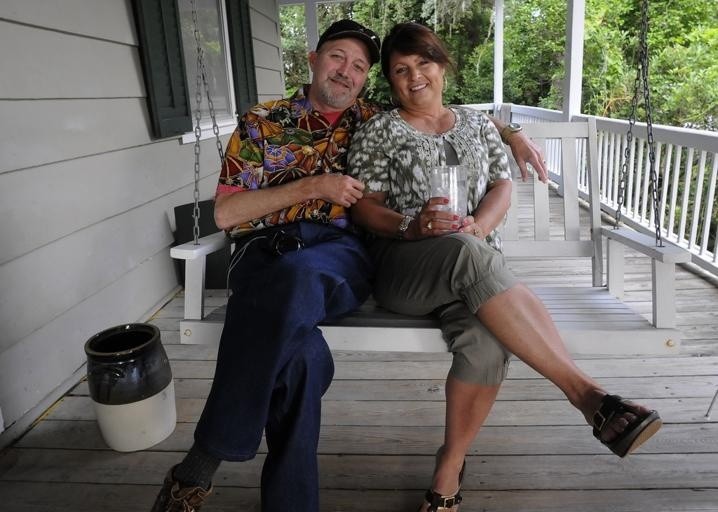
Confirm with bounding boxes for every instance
[428,165,470,234]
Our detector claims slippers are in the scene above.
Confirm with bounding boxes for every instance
[420,459,466,512]
[592,394,663,459]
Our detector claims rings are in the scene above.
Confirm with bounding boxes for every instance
[542,159,548,171]
[424,220,436,234]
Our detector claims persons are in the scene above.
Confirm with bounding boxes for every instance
[148,17,550,512]
[346,23,664,512]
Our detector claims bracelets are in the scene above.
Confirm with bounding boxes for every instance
[391,208,413,243]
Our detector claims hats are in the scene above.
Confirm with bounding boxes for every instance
[315,18,382,64]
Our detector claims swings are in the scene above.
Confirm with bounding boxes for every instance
[169,2,691,362]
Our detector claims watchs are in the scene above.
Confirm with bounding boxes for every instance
[499,115,527,140]
